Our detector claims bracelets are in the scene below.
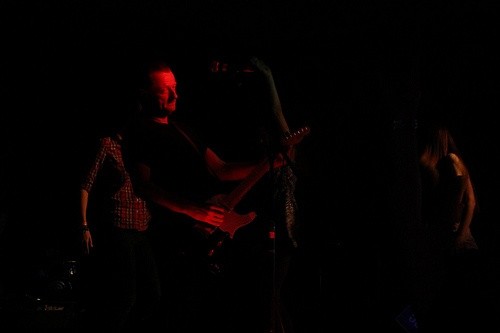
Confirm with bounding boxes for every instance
[79,224,89,233]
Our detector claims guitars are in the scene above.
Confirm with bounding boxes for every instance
[182,125,312,274]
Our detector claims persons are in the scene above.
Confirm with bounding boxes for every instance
[77,60,288,329]
[399,96,479,305]
